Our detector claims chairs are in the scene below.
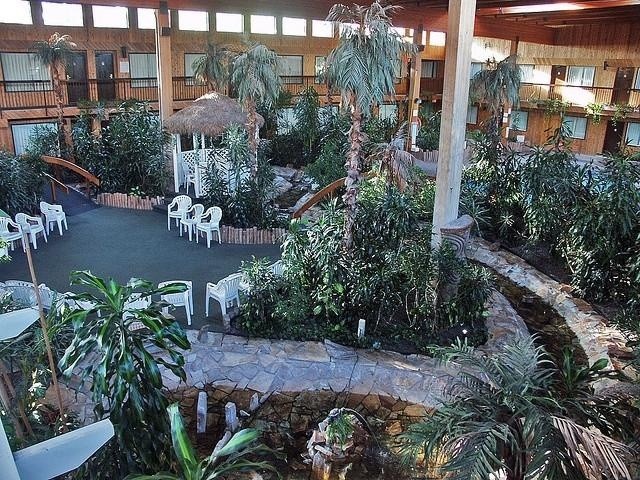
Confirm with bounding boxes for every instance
[0,272,244,324]
[166,159,224,249]
[267,260,287,275]
[0,201,68,259]
